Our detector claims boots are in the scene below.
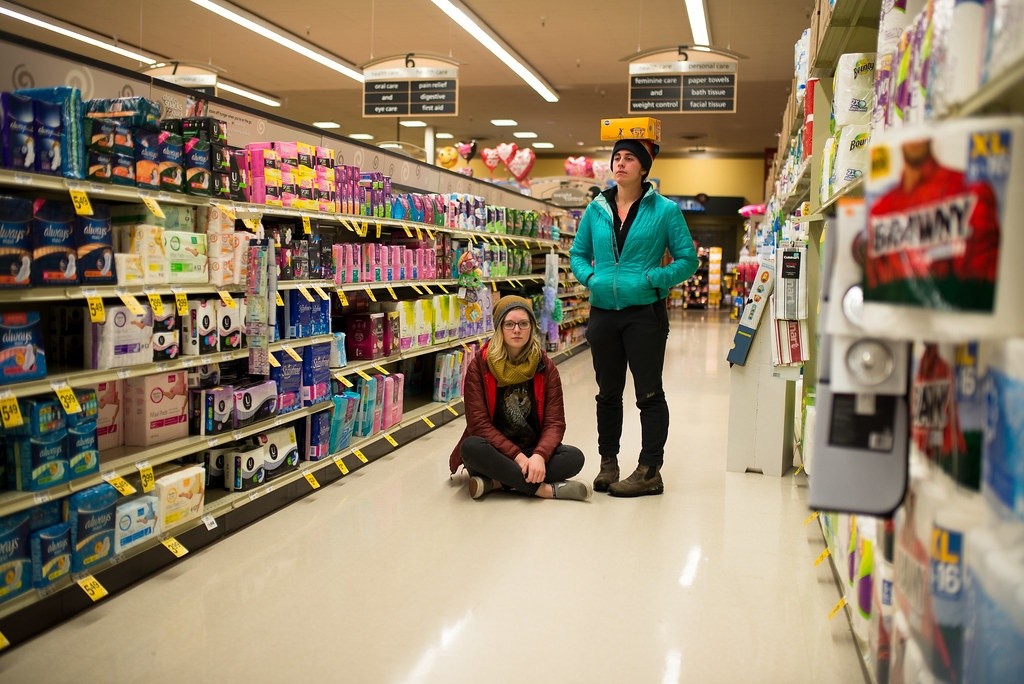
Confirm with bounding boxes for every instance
[607,463,663,498]
[593,455,620,491]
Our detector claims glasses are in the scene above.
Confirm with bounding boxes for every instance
[502,319,531,330]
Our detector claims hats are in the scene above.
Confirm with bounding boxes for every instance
[492,295,537,333]
[610,140,660,181]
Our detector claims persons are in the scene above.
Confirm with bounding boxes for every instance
[569,139,698,497]
[189,98,207,117]
[448,296,592,500]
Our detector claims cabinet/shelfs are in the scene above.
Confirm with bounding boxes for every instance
[0,167,590,653]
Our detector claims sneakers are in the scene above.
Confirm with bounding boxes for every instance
[468,476,493,499]
[551,479,593,500]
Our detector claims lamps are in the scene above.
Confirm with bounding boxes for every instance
[0,0,282,108]
[684,0,713,48]
[191,0,363,83]
[430,0,561,103]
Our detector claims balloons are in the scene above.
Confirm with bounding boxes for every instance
[459,140,477,161]
[565,156,613,180]
[496,142,518,166]
[507,148,536,183]
[480,147,499,169]
[439,146,457,168]
